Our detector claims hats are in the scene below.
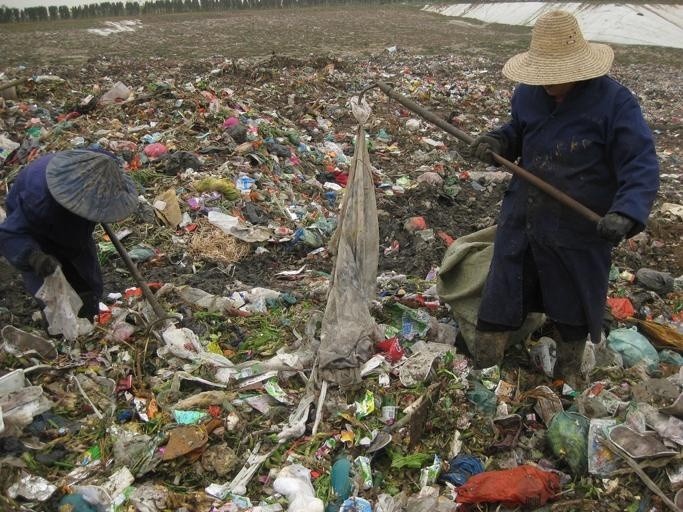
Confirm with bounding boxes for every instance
[500,9,616,88]
[43,148,140,226]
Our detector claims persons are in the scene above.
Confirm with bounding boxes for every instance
[464,9,660,392]
[0,143,138,339]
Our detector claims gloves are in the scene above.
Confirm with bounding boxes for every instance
[29,250,62,281]
[467,136,505,163]
[594,213,636,243]
